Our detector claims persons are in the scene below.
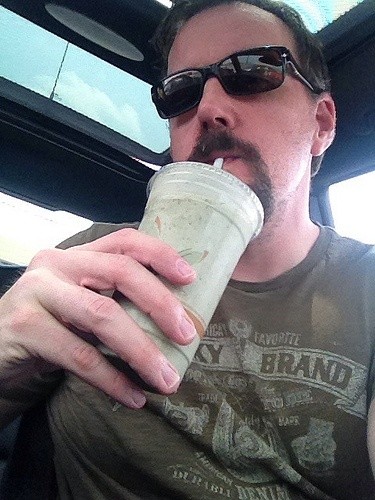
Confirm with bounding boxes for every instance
[0,0,374,500]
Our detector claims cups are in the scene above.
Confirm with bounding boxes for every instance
[94,165,266,396]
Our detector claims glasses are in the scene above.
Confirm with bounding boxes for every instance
[150,45,326,119]
